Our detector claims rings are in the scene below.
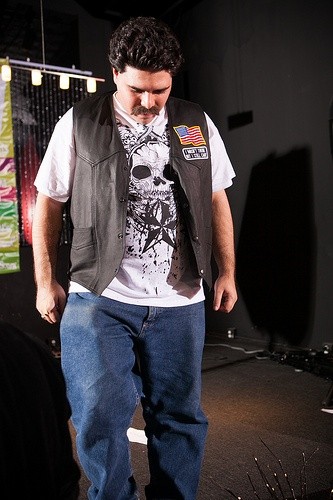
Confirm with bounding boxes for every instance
[42,315,46,319]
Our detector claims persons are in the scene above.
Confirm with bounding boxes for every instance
[32,16,237,500]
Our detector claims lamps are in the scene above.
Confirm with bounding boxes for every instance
[1,65,106,93]
